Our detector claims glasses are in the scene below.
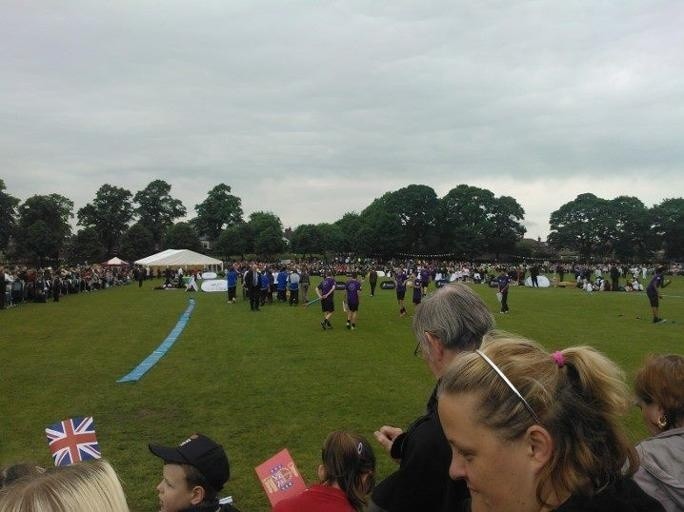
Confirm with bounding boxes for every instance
[414,330,441,360]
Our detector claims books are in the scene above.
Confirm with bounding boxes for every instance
[251,449,305,506]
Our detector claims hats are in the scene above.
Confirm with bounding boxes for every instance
[149,434,229,491]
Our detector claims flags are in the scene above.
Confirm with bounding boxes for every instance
[44,416,100,467]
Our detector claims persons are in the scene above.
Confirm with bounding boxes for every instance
[0,457,129,511]
[147,433,237,512]
[395,259,684,292]
[227,259,377,312]
[434,329,648,511]
[345,272,362,329]
[395,267,423,318]
[498,269,510,314]
[647,266,672,323]
[362,281,500,512]
[620,352,683,512]
[270,429,379,511]
[314,272,336,330]
[0,263,183,309]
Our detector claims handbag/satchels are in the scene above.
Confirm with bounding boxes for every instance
[289,284,298,289]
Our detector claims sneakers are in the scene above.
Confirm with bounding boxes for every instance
[654,318,666,324]
[346,320,355,330]
[400,311,407,317]
[321,319,333,330]
